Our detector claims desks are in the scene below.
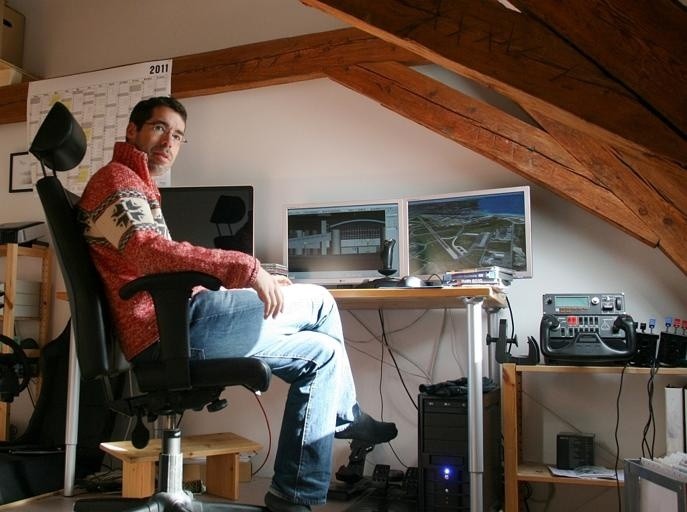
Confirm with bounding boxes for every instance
[56,288,506,511]
[499,364,687,511]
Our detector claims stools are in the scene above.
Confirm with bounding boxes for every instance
[99,432,261,500]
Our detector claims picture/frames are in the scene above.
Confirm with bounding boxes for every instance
[9,152,33,192]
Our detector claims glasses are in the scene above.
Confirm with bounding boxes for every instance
[140,122,187,146]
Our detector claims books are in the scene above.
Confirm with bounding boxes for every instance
[444,264,515,295]
[547,461,619,480]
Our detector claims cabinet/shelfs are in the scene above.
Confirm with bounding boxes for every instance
[0,242,52,440]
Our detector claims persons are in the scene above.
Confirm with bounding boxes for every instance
[78,95,400,512]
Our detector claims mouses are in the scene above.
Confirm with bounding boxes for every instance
[397,275,427,287]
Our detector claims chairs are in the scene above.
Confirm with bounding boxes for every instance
[28,103,272,512]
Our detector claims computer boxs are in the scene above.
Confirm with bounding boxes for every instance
[418,386,505,512]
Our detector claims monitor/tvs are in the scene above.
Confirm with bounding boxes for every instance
[155,185,255,257]
[404,185,535,279]
[282,197,405,287]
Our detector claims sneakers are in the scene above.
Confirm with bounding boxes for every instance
[263,490,313,512]
[333,410,398,444]
